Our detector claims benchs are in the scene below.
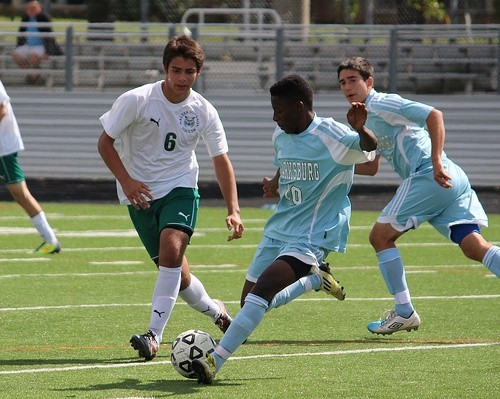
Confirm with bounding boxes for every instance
[0,41,500,95]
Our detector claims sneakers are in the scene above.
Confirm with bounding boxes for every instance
[315,263,345,300]
[34,241,60,254]
[367,309,420,336]
[211,299,247,344]
[129,330,159,360]
[191,360,213,386]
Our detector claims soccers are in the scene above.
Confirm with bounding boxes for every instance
[170,329,217,379]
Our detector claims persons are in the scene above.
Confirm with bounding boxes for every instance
[97,36,248,361]
[190,73,379,385]
[0,80,61,253]
[12,0,62,85]
[336,56,500,337]
